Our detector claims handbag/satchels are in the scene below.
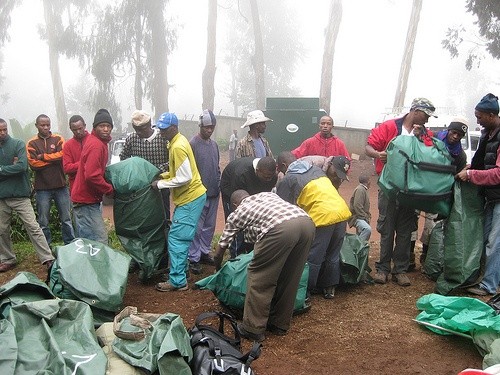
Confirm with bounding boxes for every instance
[186,310,261,375]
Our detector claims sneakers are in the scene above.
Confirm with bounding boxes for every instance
[373,269,387,284]
[189,260,203,275]
[392,271,411,285]
[200,252,215,266]
[155,280,189,292]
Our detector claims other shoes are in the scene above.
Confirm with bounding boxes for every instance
[267,323,288,336]
[467,285,488,296]
[237,322,265,342]
[323,284,336,299]
[47,261,52,269]
[0,262,15,273]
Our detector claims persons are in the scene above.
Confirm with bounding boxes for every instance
[147,113,208,291]
[187,109,222,274]
[228,130,241,162]
[274,150,352,297]
[289,115,352,186]
[118,109,173,271]
[236,108,274,157]
[218,156,279,260]
[363,97,436,284]
[0,118,56,273]
[69,108,117,248]
[25,114,76,252]
[277,154,350,191]
[63,114,91,202]
[402,120,472,275]
[348,175,373,272]
[455,90,500,296]
[212,187,315,344]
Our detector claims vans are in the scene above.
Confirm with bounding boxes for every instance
[460,130,482,166]
[111,138,127,164]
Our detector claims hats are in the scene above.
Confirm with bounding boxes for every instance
[92,109,113,128]
[447,122,468,137]
[131,112,151,127]
[241,110,274,129]
[332,155,350,182]
[474,93,499,115]
[411,97,438,119]
[198,110,216,128]
[155,112,178,129]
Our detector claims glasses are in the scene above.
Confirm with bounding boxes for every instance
[418,105,436,112]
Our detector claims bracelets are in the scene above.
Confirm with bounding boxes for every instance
[466,169,470,182]
